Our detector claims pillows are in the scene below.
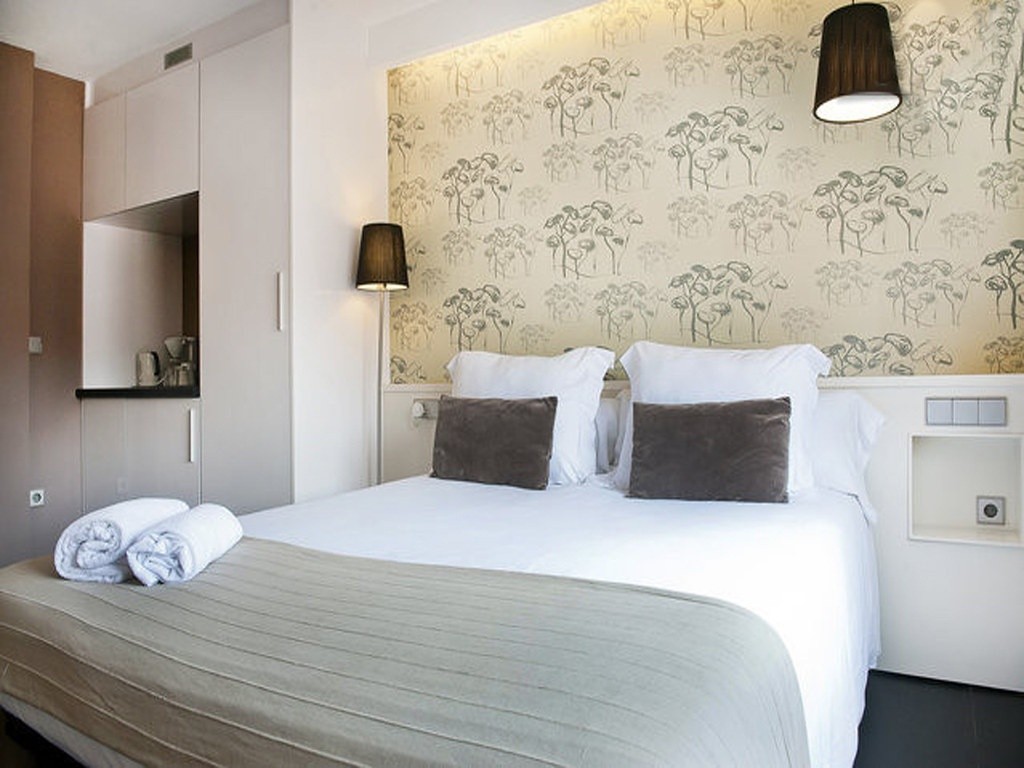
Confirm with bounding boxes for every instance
[628,396,791,504]
[430,393,558,489]
[614,389,866,496]
[611,340,824,491]
[447,346,616,488]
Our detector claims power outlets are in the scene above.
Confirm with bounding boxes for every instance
[29,336,43,353]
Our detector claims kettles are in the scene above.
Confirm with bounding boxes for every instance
[134,347,161,387]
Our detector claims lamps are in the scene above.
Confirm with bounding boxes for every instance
[356,223,411,483]
[812,0,903,123]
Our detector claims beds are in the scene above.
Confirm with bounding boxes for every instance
[0,474,880,768]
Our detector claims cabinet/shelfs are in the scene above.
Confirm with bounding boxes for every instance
[84,62,200,236]
[81,398,201,514]
[200,24,287,516]
